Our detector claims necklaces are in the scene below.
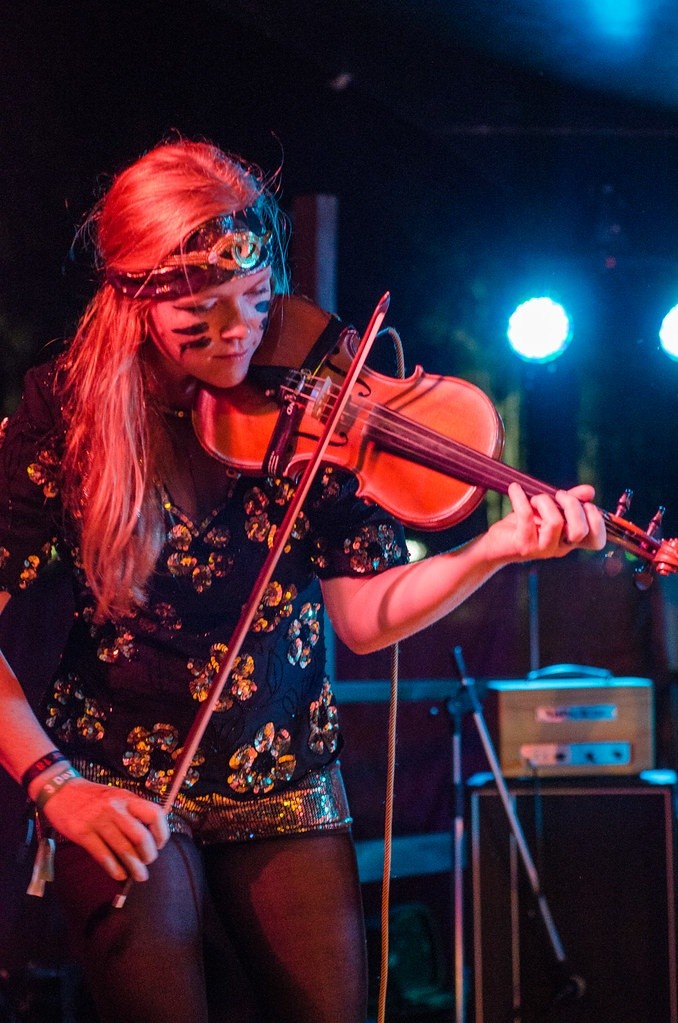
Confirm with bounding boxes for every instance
[152,398,193,424]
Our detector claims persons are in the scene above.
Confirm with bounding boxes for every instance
[0,141,606,1023]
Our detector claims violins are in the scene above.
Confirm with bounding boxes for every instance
[187,293,678,593]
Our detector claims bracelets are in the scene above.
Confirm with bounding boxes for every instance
[21,751,84,897]
